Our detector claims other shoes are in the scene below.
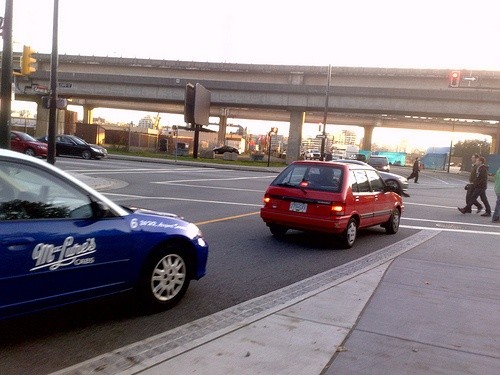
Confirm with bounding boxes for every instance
[492,215,500,223]
[480,212,491,217]
[477,205,483,213]
[466,209,471,213]
[458,207,466,214]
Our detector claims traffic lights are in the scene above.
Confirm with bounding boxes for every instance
[450,71,460,88]
[21,45,37,77]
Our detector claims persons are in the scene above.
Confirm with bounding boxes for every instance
[458,157,491,217]
[491,166,500,223]
[407,157,421,183]
[466,153,483,214]
[326,152,332,161]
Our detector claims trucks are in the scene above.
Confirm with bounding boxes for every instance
[358,150,407,167]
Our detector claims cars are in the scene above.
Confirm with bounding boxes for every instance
[329,160,409,191]
[212,145,239,155]
[260,161,404,248]
[37,134,108,160]
[11,130,49,157]
[305,148,321,160]
[0,150,208,327]
[368,156,390,173]
